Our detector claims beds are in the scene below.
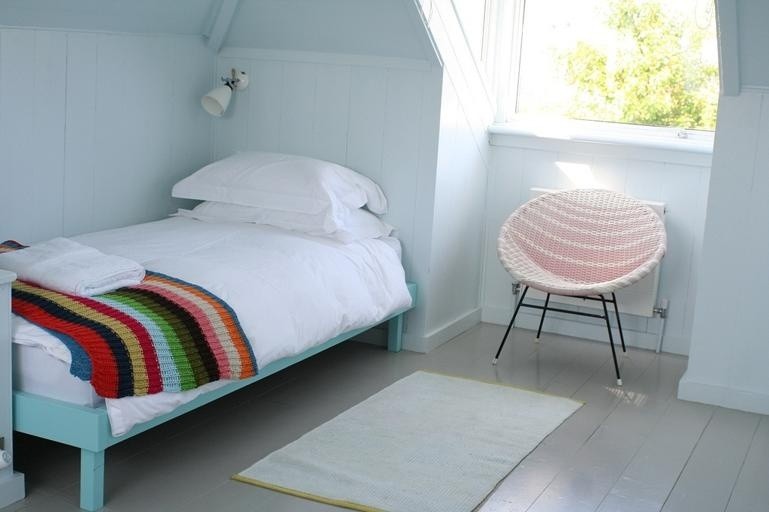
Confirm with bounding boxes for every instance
[0,212,418,510]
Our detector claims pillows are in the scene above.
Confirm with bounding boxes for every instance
[178,202,397,244]
[172,151,389,233]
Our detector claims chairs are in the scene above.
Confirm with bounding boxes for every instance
[492,188,668,386]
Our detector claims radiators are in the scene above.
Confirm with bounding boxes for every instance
[511,188,668,320]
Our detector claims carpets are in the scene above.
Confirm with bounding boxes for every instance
[231,370,587,512]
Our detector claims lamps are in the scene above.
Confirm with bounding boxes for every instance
[201,67,249,117]
